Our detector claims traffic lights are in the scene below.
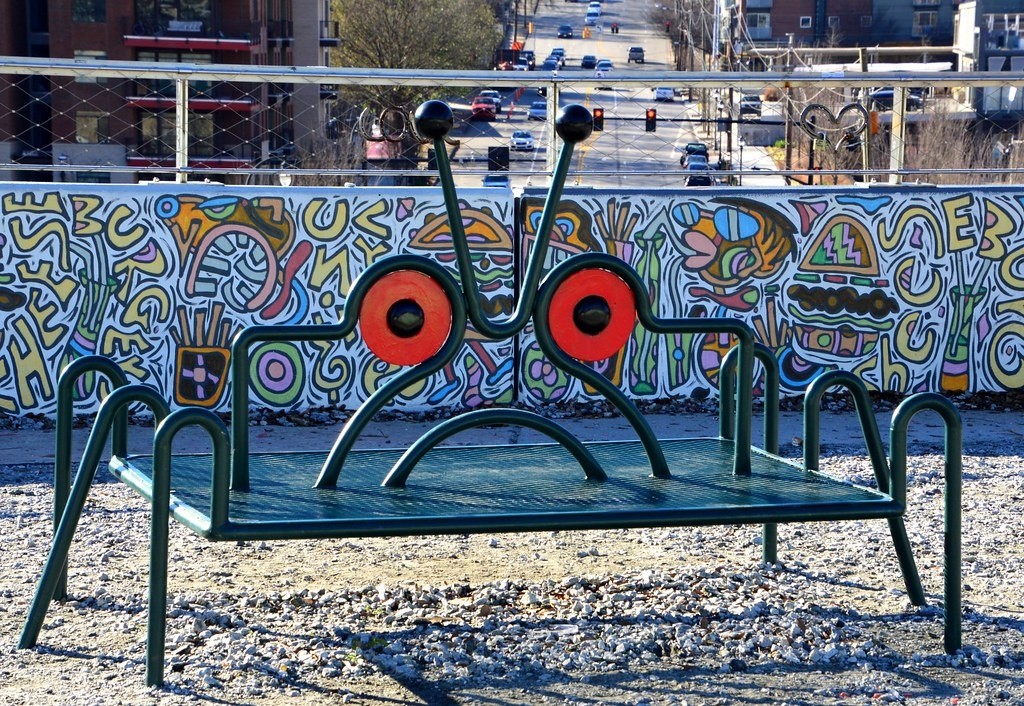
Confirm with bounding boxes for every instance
[665,21,671,34]
[646,109,659,132]
[611,24,619,34]
[593,106,605,132]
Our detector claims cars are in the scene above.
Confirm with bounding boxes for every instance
[739,94,765,117]
[483,175,512,191]
[527,102,548,121]
[627,46,646,63]
[494,49,537,72]
[651,85,675,101]
[581,55,597,69]
[479,90,504,114]
[538,47,567,93]
[679,141,714,186]
[469,96,497,121]
[585,1,602,25]
[557,24,574,39]
[510,130,534,151]
[595,59,617,92]
[856,88,925,112]
